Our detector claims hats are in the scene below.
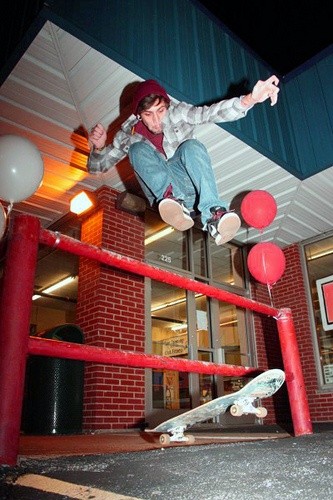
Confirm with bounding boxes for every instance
[128,79,171,115]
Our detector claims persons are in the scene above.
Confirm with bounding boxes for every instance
[85,74,279,247]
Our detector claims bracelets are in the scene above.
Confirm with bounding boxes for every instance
[92,144,107,151]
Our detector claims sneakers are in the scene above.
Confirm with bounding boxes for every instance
[206,205,242,245]
[159,196,195,233]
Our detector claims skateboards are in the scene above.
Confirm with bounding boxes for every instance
[145,368,286,446]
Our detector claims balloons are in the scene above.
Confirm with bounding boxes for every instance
[246,242,286,288]
[0,135,45,210]
[241,190,278,232]
